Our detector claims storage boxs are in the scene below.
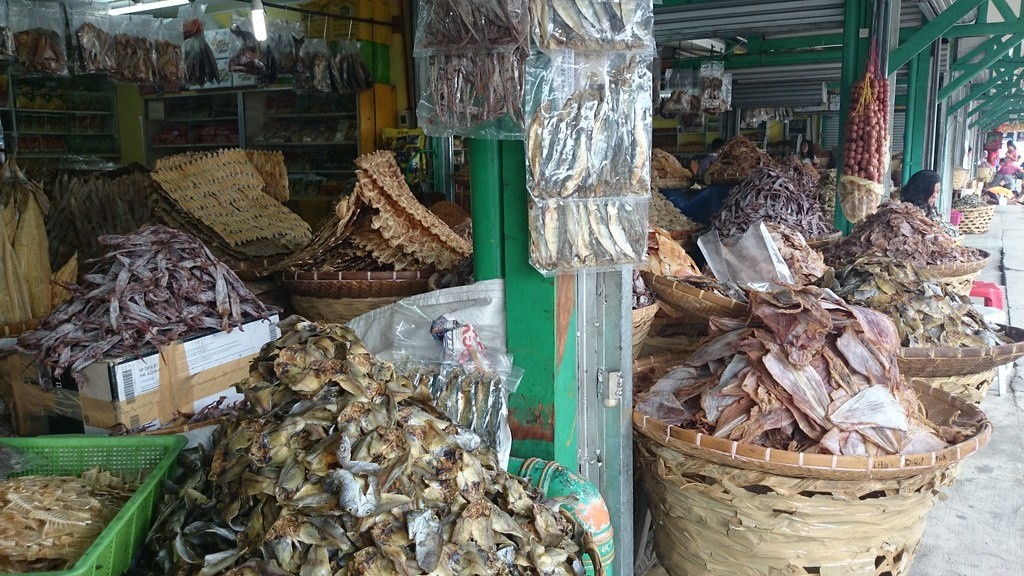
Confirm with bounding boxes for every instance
[73,306,283,438]
[172,28,391,91]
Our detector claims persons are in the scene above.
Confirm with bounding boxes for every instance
[981,138,1024,197]
[697,137,725,176]
[903,170,941,216]
[800,139,819,169]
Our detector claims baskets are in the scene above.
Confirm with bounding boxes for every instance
[650,226,702,240]
[805,230,842,251]
[632,302,659,361]
[896,322,1024,376]
[825,246,990,276]
[955,206,993,235]
[706,171,751,185]
[293,296,400,324]
[640,270,752,323]
[655,177,693,189]
[0,434,189,576]
[285,264,434,297]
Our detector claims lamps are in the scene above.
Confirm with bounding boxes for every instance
[108,0,194,17]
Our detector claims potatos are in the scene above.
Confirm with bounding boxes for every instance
[844,73,890,183]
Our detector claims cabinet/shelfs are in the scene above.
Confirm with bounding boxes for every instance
[0,66,153,171]
[139,62,398,201]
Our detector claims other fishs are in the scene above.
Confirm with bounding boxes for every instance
[0,1,1015,575]
